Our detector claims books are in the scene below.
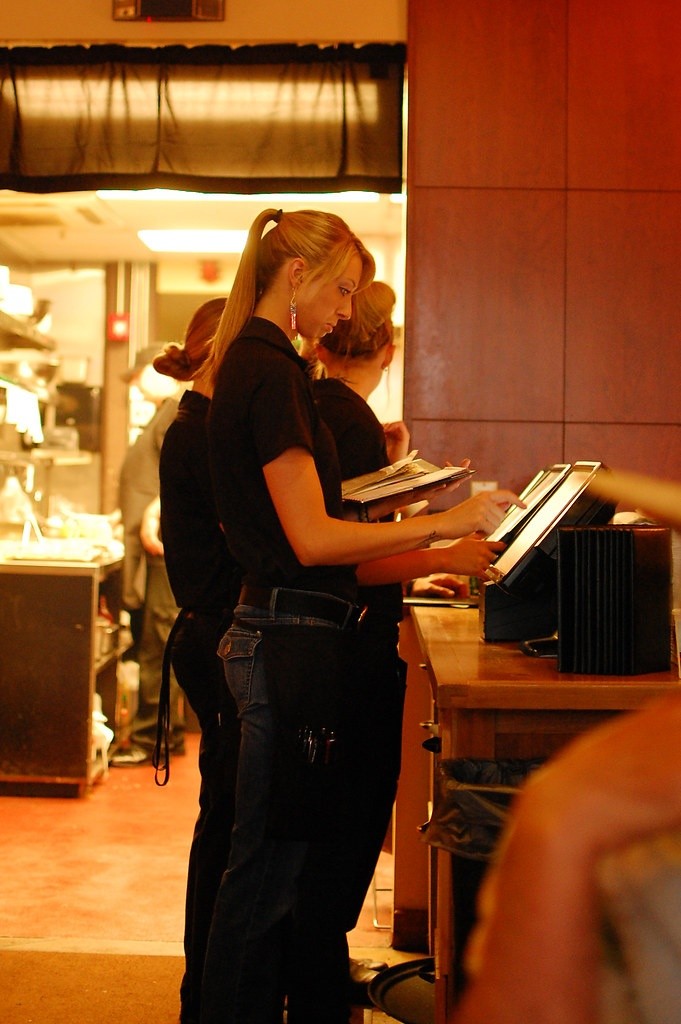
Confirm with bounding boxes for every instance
[336,458,479,503]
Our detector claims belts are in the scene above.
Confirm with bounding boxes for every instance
[183,610,228,627]
[240,584,374,624]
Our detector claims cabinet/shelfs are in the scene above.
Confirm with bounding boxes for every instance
[411,603,681,1024]
[0,550,134,799]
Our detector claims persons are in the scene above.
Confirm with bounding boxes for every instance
[115,210,527,1024]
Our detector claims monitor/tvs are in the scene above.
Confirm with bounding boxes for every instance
[475,461,620,601]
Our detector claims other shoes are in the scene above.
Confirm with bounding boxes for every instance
[350,959,390,984]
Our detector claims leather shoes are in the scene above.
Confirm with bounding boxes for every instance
[112,740,185,767]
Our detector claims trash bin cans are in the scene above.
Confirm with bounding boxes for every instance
[436,755,552,1024]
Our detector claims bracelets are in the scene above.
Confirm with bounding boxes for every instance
[356,505,369,523]
